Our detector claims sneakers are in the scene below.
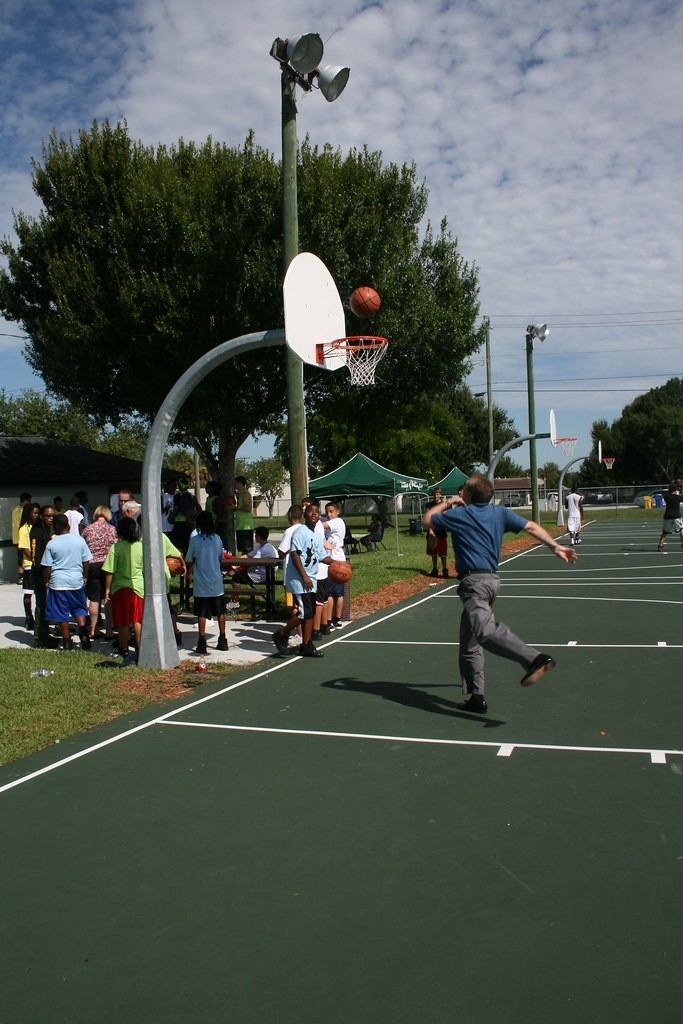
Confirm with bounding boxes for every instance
[226,600,240,610]
[300,641,325,658]
[273,628,290,655]
[441,569,450,579]
[430,569,439,578]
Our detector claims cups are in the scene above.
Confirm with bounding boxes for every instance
[235,551,243,557]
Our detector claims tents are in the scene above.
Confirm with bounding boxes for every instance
[309,452,472,556]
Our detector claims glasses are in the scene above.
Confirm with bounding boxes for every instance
[41,514,55,518]
[120,499,130,503]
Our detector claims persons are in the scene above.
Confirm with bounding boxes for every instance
[185,511,229,653]
[271,496,345,658]
[82,489,187,656]
[657,479,683,551]
[565,487,584,545]
[160,476,254,555]
[12,490,93,651]
[226,526,280,610]
[421,474,577,712]
[336,513,383,552]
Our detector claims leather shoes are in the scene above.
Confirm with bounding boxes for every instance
[458,697,487,713]
[520,655,555,687]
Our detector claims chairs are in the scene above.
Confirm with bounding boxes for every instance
[373,528,387,551]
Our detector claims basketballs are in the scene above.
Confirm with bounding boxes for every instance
[350,286,381,318]
[328,561,351,584]
[166,557,183,576]
[223,496,236,508]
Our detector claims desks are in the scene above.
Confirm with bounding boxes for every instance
[180,558,283,612]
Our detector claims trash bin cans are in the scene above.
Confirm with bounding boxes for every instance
[655,494,663,507]
[408,519,422,534]
[643,496,652,510]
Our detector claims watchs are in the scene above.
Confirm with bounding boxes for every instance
[442,499,449,507]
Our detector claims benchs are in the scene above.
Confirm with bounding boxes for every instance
[173,577,283,595]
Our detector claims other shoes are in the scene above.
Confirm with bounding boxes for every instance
[176,631,182,645]
[570,538,582,545]
[312,617,341,640]
[658,545,664,552]
[25,615,142,661]
[195,640,207,654]
[216,640,228,650]
